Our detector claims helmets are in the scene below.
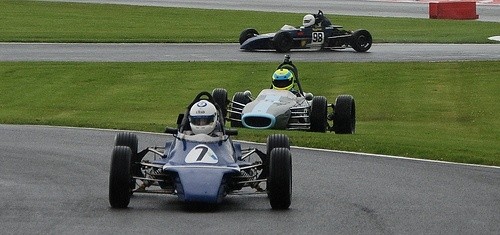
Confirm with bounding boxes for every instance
[272,68,294,90]
[303,14,315,27]
[188,100,217,135]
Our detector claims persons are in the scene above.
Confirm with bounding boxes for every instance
[188,100,220,138]
[302,14,315,29]
[269,68,300,97]
